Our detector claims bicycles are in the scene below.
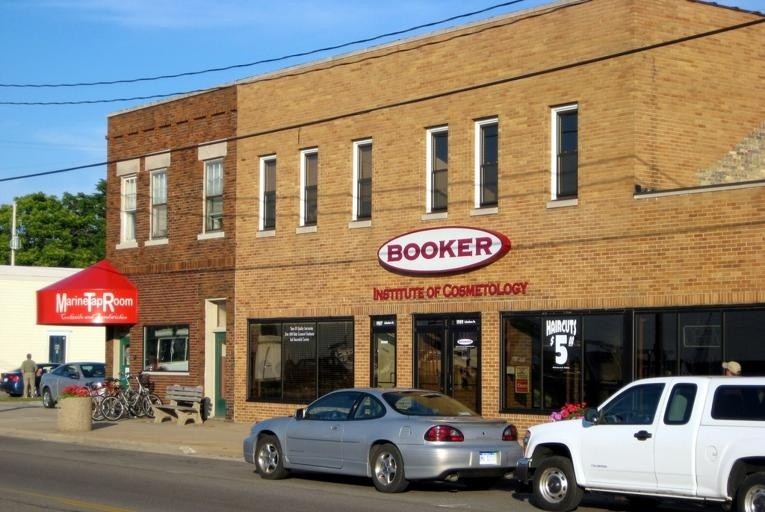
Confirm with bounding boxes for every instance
[82,368,163,422]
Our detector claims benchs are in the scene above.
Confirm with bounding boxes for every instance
[148,383,205,427]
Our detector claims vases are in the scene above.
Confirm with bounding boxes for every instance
[54,396,93,433]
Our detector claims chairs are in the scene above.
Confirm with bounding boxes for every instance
[666,393,689,422]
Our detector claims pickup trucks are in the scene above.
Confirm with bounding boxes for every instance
[509,372,764,512]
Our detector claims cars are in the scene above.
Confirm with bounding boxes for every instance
[287,355,349,381]
[242,384,525,496]
[38,359,105,411]
[0,362,76,398]
[580,349,624,387]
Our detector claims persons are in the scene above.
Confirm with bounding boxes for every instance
[721,360,742,376]
[20,353,38,398]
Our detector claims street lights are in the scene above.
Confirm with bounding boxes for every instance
[7,233,22,266]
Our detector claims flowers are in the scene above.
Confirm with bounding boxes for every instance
[57,383,90,400]
[548,399,587,423]
